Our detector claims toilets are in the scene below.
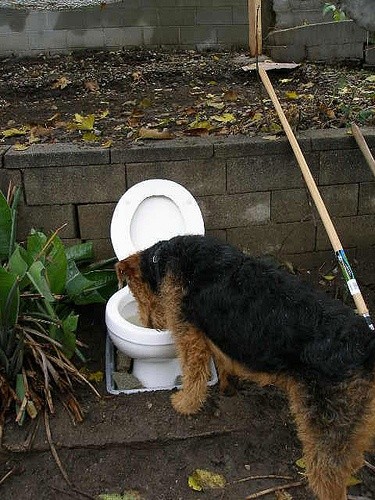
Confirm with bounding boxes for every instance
[104,178,219,393]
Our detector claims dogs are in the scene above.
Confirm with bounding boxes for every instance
[115,234,375,500]
